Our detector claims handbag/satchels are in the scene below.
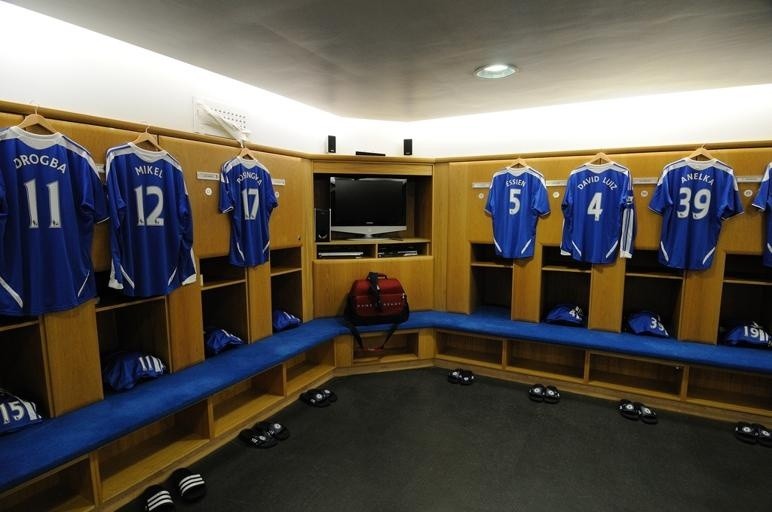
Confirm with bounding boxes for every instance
[342,272,410,353]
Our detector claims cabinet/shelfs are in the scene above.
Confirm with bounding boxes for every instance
[1,101,772,418]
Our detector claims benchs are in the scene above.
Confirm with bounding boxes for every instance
[0,313,771,512]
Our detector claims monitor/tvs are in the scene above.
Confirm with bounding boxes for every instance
[329,177,408,240]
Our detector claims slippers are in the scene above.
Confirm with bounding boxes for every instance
[734,420,759,445]
[459,371,474,385]
[751,422,772,447]
[141,485,177,512]
[307,388,338,403]
[632,401,659,425]
[527,383,545,403]
[543,384,562,405]
[250,422,290,448]
[167,467,207,502]
[448,368,463,384]
[299,392,331,408]
[239,420,277,444]
[617,398,642,422]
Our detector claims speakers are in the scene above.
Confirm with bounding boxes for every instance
[328,136,336,153]
[404,139,412,155]
[315,208,331,243]
